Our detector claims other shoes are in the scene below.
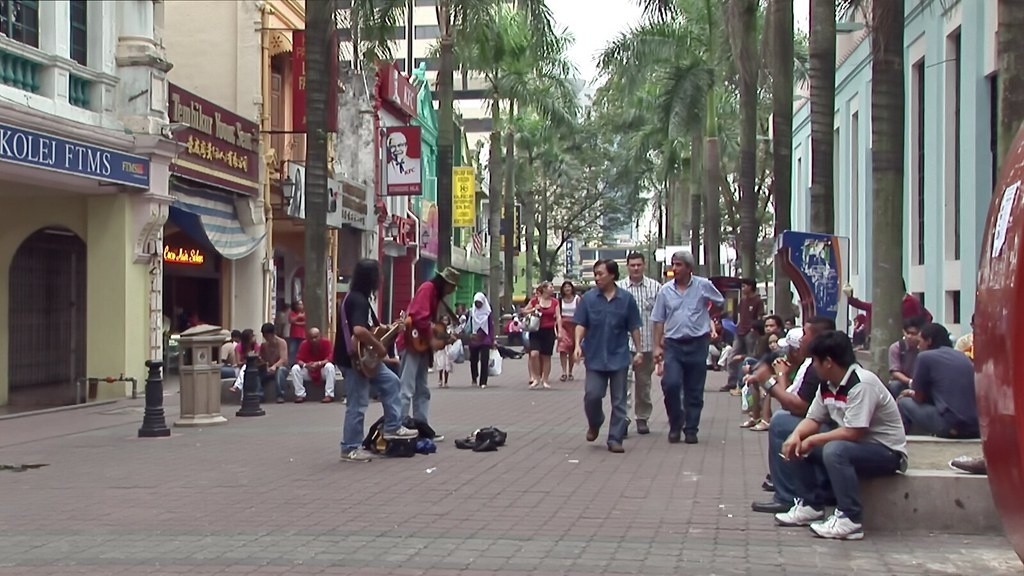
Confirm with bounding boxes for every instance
[472,381,477,386]
[294,397,304,402]
[555,374,698,451]
[528,382,539,389]
[481,385,487,389]
[276,396,284,403]
[323,396,334,402]
[433,435,444,442]
[428,368,434,373]
[950,455,987,474]
[445,384,448,388]
[229,387,237,392]
[529,379,533,385]
[542,382,550,389]
[438,382,443,386]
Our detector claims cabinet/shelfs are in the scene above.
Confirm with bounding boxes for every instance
[162,333,185,376]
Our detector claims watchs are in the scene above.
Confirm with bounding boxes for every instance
[764,377,778,390]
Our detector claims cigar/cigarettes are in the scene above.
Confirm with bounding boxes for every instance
[778,453,791,461]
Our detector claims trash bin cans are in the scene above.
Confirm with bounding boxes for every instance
[172,324,228,426]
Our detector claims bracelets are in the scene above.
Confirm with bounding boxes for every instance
[303,363,306,368]
[908,379,913,389]
[776,372,784,377]
[319,361,321,367]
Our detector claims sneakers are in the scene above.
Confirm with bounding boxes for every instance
[706,368,864,540]
[340,445,372,463]
[383,424,419,439]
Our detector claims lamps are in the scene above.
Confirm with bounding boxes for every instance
[162,122,190,139]
[272,176,295,206]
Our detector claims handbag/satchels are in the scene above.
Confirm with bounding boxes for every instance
[362,414,436,457]
[449,339,465,364]
[520,312,541,332]
[740,381,754,414]
[487,348,502,377]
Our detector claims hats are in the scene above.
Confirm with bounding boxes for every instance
[435,267,462,288]
[777,328,804,347]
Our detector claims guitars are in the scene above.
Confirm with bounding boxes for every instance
[405,315,471,353]
[350,310,408,378]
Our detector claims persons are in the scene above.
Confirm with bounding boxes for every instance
[650,251,723,443]
[706,277,987,540]
[218,251,663,453]
[333,259,418,462]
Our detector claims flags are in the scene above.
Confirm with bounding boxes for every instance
[473,231,480,254]
